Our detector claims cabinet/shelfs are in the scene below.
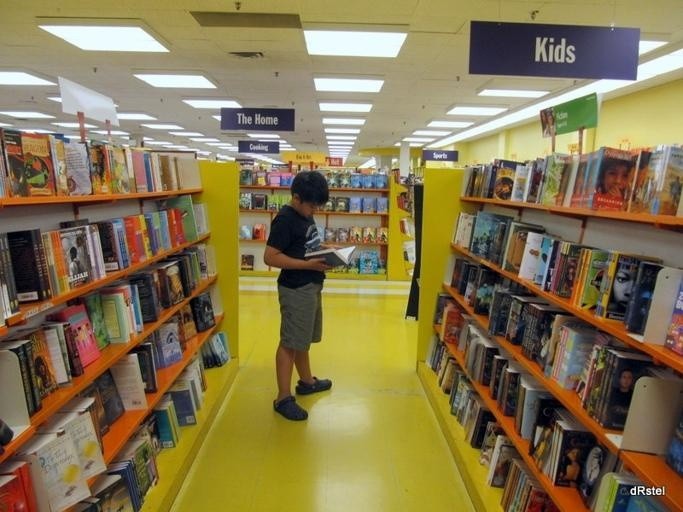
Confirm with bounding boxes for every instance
[431,196,681,511]
[1,184,223,511]
[238,184,387,275]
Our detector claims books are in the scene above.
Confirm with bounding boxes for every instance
[426,142,682,508]
[1,127,232,510]
[240,162,419,283]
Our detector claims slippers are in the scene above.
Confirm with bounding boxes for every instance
[295,376,332,395]
[273,397,308,421]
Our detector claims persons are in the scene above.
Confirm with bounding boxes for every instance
[265,170,343,422]
